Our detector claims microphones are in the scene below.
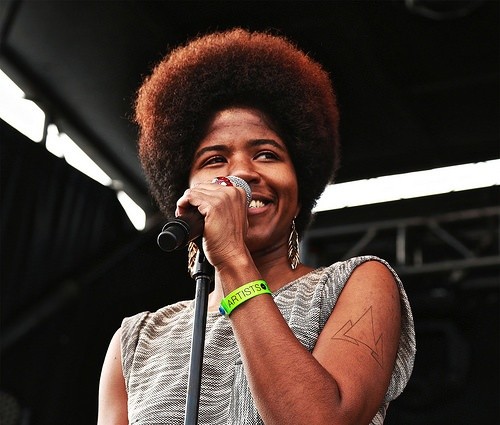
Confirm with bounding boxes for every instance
[156,175,253,252]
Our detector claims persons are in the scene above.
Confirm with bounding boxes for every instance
[97,30,416,425]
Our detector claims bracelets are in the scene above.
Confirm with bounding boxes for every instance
[219,280,271,319]
